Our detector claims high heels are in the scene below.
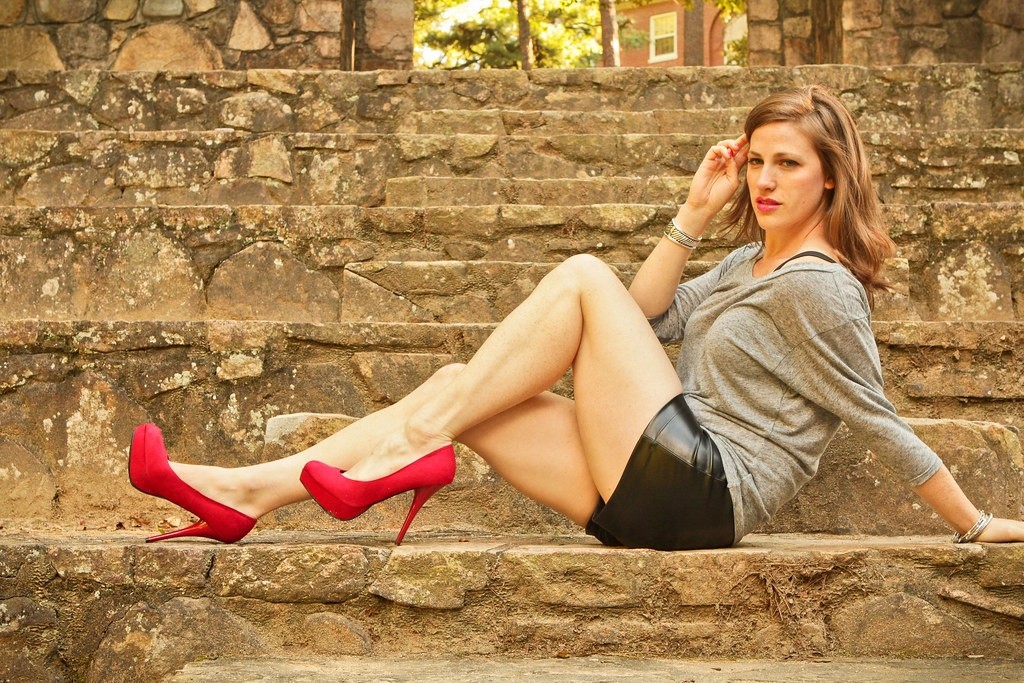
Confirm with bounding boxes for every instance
[129,424,257,544]
[299,444,456,546]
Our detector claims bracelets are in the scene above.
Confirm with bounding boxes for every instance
[952,510,994,543]
[665,220,700,249]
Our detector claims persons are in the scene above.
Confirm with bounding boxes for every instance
[129,85,1024,551]
